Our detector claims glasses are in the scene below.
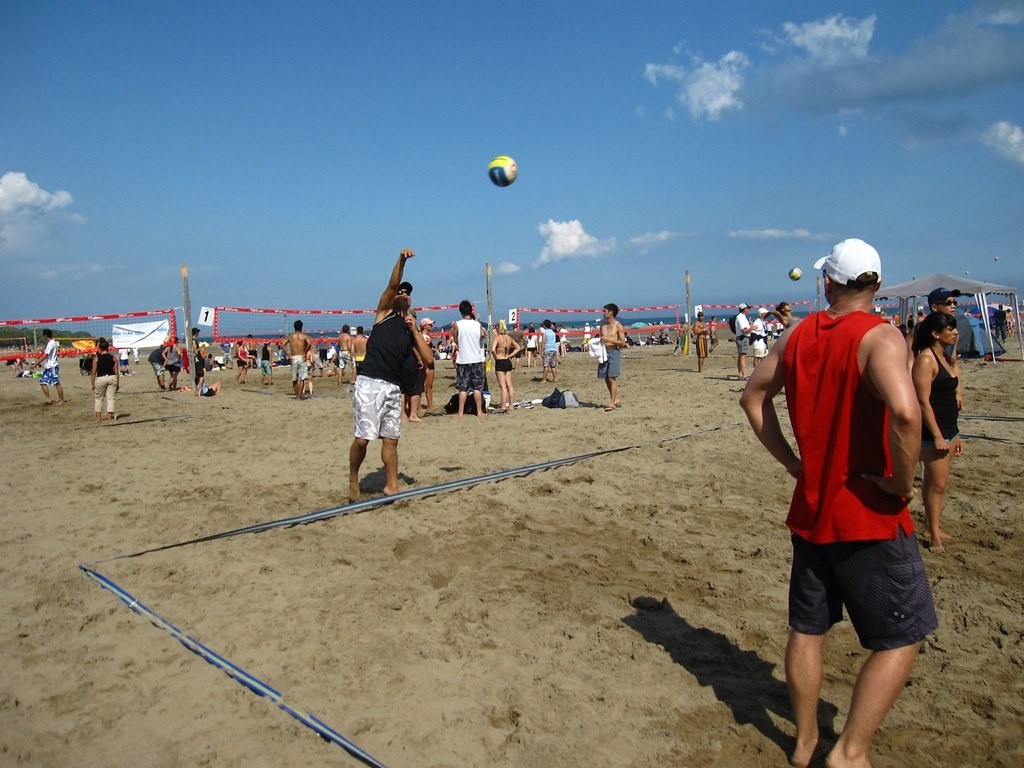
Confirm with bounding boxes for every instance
[413,315,417,318]
[942,301,957,306]
[397,290,410,296]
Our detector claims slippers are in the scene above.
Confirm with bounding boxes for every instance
[606,402,621,412]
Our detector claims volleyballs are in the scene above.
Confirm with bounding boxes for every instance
[994,256,999,261]
[488,155,517,188]
[446,355,451,359]
[789,267,802,281]
[965,271,969,274]
[326,370,333,376]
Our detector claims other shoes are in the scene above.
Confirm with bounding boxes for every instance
[738,376,742,380]
[671,351,678,356]
[425,409,441,415]
[741,376,750,380]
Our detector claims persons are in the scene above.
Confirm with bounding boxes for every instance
[348,248,435,502]
[419,318,436,412]
[401,308,424,423]
[33,329,65,406]
[147,301,1024,413]
[91,340,120,422]
[452,300,486,418]
[911,309,963,555]
[5,358,25,378]
[736,237,940,768]
[905,287,964,481]
[539,319,558,384]
[592,303,626,411]
[490,319,520,414]
[118,347,139,377]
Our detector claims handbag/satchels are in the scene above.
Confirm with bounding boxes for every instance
[542,387,579,409]
[443,394,487,415]
[280,358,291,365]
[729,314,738,334]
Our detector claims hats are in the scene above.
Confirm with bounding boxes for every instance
[698,312,703,316]
[398,282,413,292]
[420,318,436,326]
[192,328,200,332]
[585,322,589,325]
[814,237,881,287]
[758,308,770,314]
[263,337,272,344]
[407,310,416,315]
[739,303,752,310]
[928,287,960,307]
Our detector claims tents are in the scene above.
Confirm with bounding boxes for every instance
[953,313,1008,358]
[874,271,1024,367]
